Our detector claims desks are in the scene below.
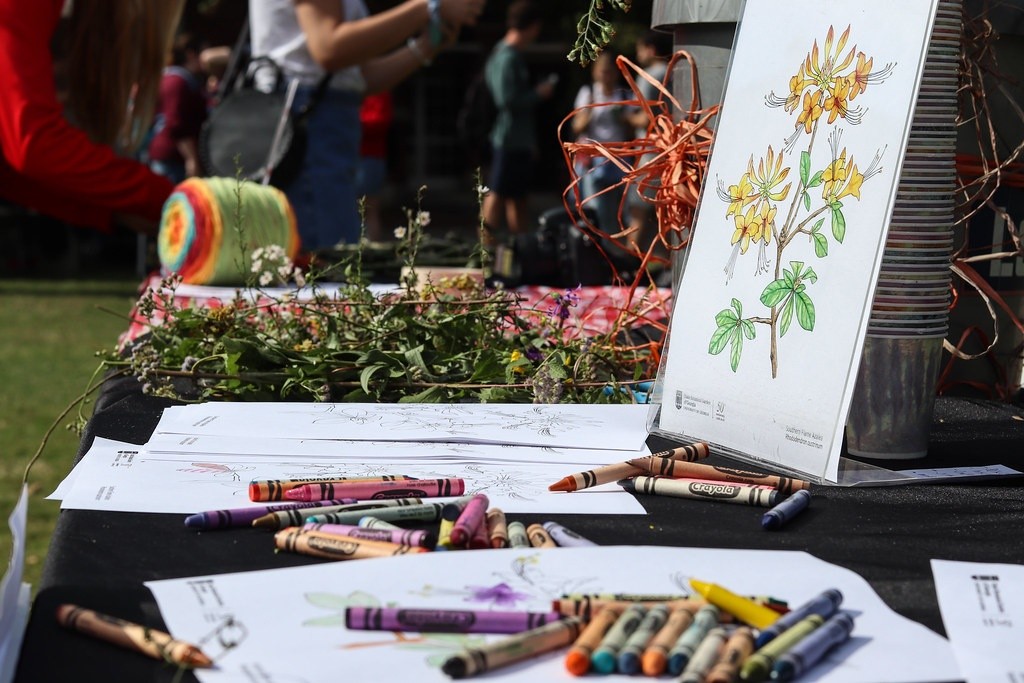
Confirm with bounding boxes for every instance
[0,276,1024,683]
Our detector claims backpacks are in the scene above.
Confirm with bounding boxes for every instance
[457,74,500,174]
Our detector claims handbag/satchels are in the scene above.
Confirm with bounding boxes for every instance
[195,57,304,181]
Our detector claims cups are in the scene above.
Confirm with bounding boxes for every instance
[846,2,962,461]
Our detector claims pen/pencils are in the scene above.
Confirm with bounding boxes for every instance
[181,438,819,559]
[344,576,857,683]
[54,603,215,669]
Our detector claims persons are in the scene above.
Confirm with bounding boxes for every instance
[248,0,480,254]
[475,0,557,296]
[568,33,672,287]
[0,0,231,281]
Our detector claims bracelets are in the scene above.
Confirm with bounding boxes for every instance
[406,37,436,77]
[427,0,442,26]
